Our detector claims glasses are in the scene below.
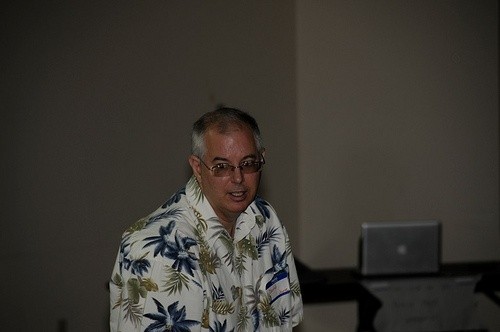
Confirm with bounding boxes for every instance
[194,150,265,176]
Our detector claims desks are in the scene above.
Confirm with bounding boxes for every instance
[294,258,500,332]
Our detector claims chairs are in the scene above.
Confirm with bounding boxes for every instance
[354,269,491,332]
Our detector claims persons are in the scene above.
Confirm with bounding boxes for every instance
[107,105,307,332]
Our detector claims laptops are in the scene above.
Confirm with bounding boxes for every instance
[360,220,442,274]
